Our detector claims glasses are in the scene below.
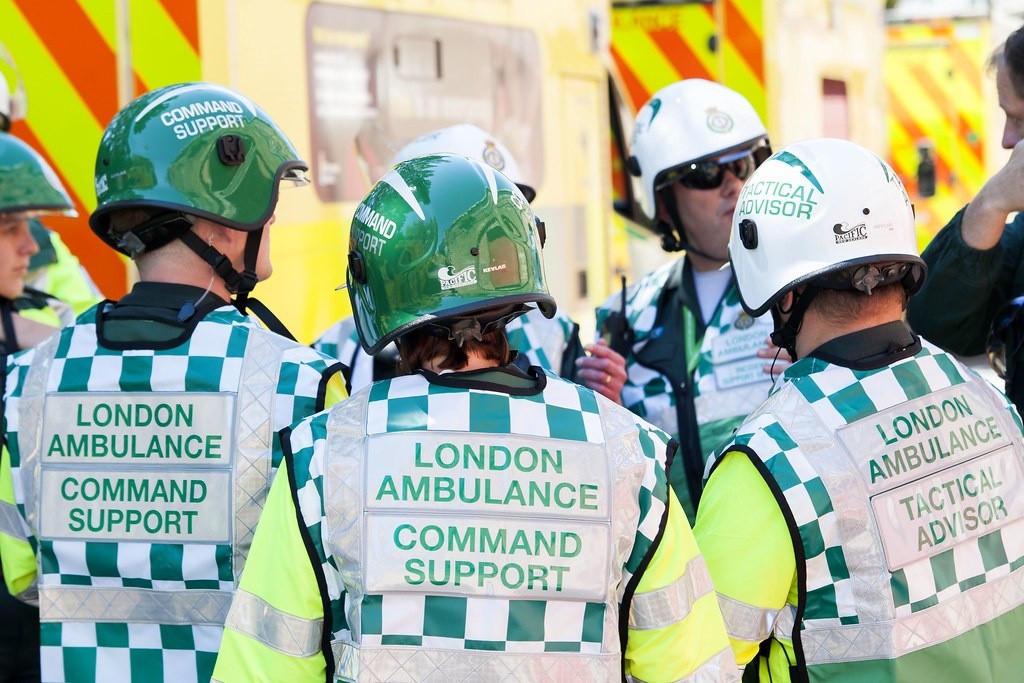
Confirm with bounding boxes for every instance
[657,147,755,199]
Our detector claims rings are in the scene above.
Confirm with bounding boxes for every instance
[606,376,611,385]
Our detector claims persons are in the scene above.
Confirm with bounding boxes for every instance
[0,25,1024,683]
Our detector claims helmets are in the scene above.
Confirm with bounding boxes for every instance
[391,124,536,208]
[728,139,926,316]
[347,154,556,353]
[88,83,309,258]
[629,80,770,220]
[0,130,73,210]
